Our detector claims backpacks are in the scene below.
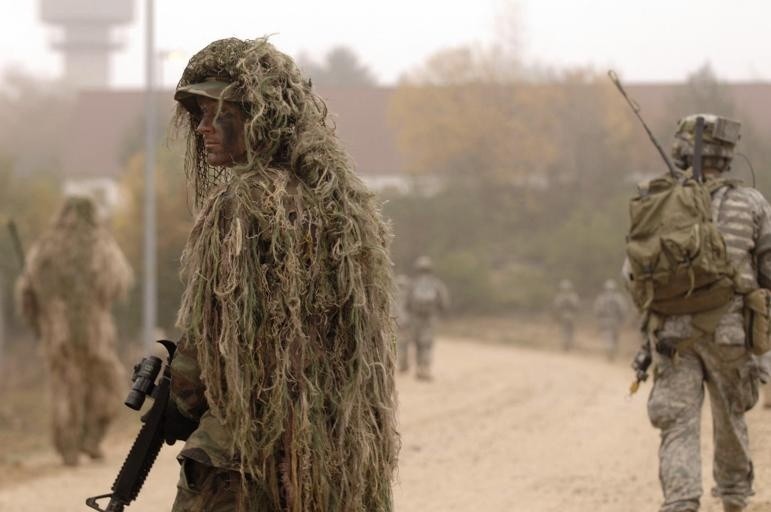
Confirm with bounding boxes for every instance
[626,177,755,315]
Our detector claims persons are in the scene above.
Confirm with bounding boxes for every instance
[151,35,405,510]
[623,113,771,510]
[13,176,139,469]
[408,253,452,383]
[390,269,414,379]
[551,277,583,355]
[593,275,632,368]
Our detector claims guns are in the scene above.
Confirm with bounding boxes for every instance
[631,338,651,392]
[86,340,199,511]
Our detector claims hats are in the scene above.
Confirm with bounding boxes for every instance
[172,56,254,102]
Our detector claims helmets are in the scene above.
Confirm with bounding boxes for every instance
[672,113,740,161]
